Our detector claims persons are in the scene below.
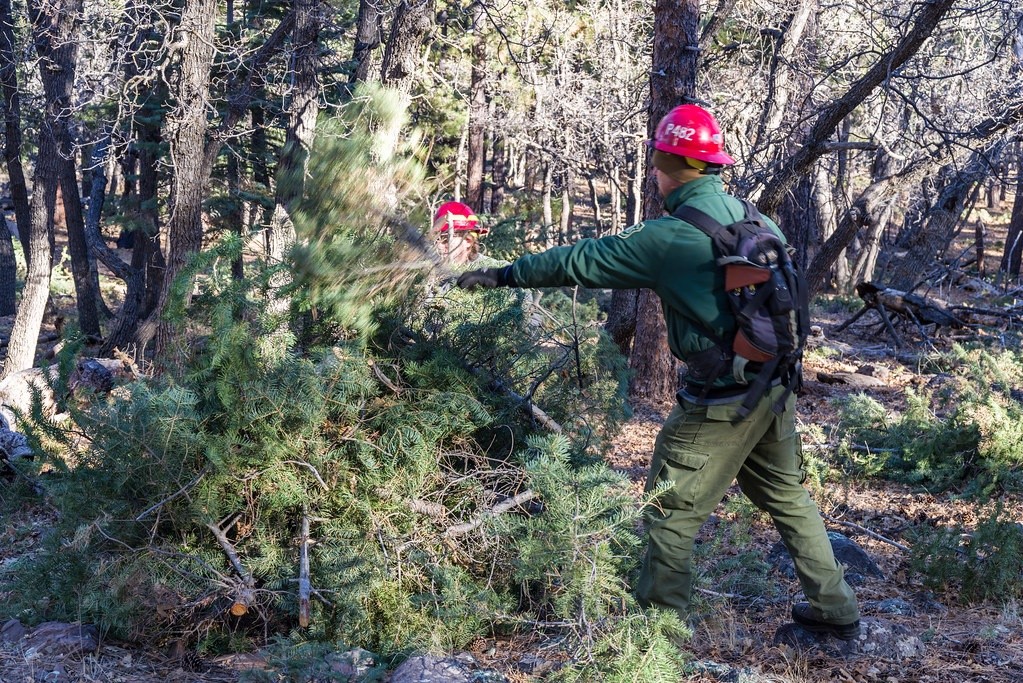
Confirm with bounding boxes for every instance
[426,201,541,352]
[456,104,864,644]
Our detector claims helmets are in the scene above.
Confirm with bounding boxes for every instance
[644,103,735,164]
[434,198,488,234]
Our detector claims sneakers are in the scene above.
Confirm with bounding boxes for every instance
[791,601,862,641]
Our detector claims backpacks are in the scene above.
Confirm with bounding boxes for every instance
[669,199,812,380]
